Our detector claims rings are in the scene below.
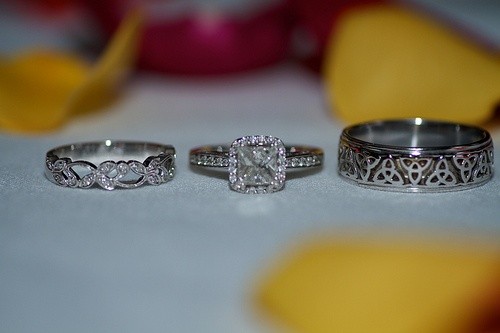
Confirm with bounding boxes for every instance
[190,134,324,195]
[338,115,495,194]
[44,140,177,191]
